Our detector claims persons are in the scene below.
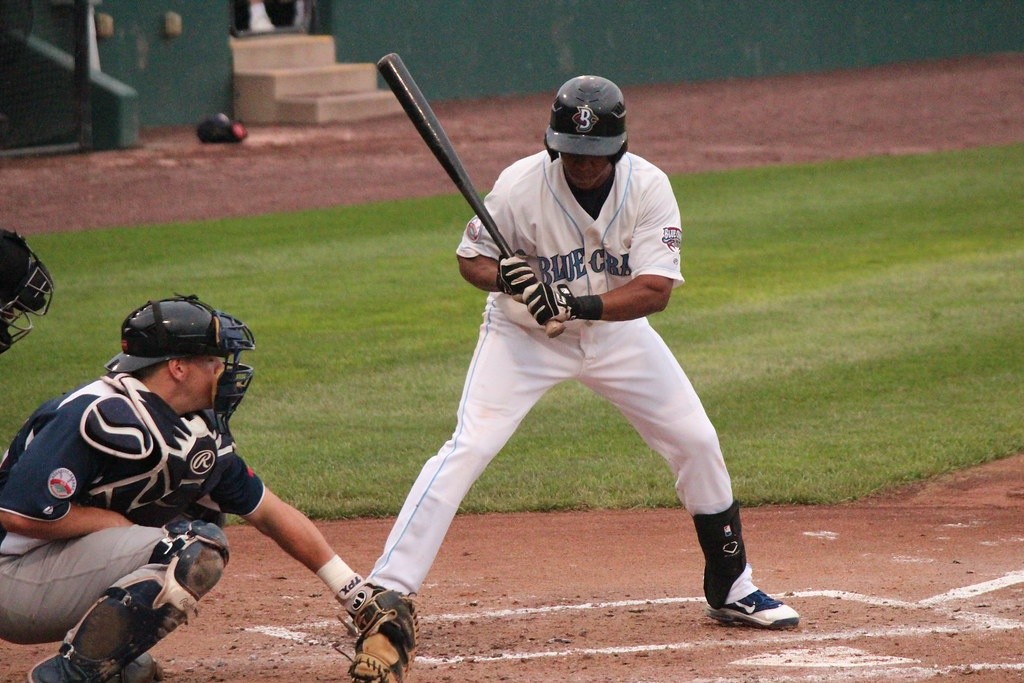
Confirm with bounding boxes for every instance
[347,75,800,636]
[0,294,414,683]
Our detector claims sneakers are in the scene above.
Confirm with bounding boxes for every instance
[705,589,801,631]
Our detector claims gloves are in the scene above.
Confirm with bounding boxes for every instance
[496,249,604,326]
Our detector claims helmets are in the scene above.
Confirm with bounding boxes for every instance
[103,296,244,374]
[543,75,629,164]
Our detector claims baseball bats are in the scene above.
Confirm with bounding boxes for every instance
[376,52,566,340]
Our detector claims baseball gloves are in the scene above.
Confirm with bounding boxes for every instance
[332,582,424,683]
